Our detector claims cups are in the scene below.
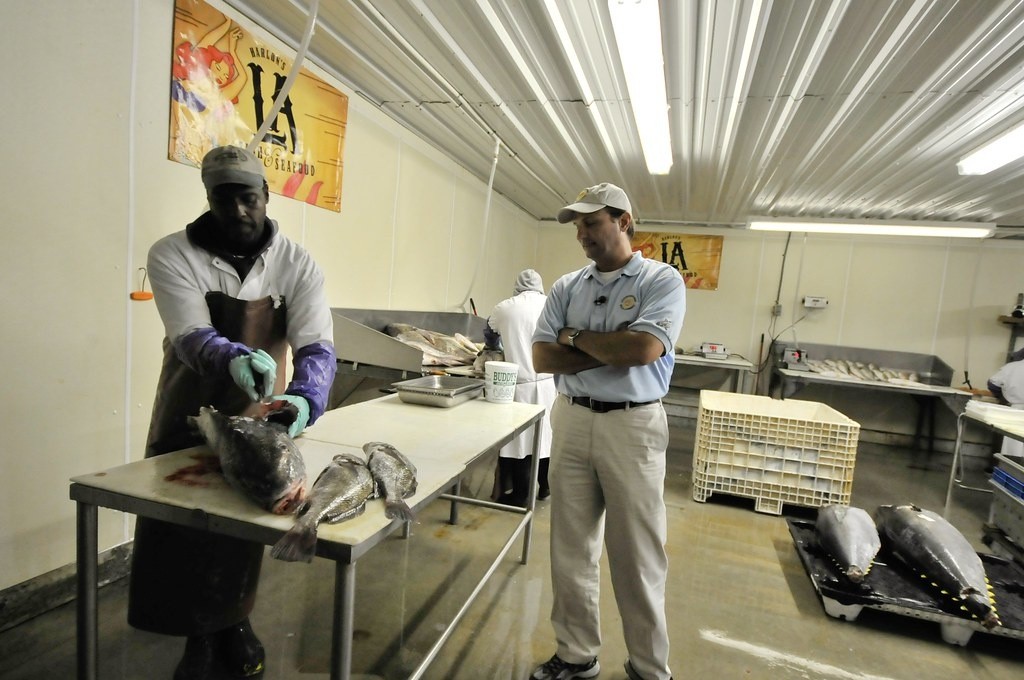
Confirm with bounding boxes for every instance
[485,361,519,404]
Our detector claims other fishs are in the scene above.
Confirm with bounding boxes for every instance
[872,503,1002,632]
[270,453,375,563]
[473,347,504,376]
[385,322,480,366]
[806,359,926,387]
[363,442,422,525]
[814,506,882,583]
[185,401,306,515]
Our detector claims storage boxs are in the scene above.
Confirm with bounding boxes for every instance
[692,390,861,515]
[986,452,1024,550]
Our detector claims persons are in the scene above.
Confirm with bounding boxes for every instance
[529,183,686,680]
[483,269,558,508]
[127,147,337,680]
[987,349,1024,456]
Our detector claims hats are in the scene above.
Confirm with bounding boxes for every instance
[556,182,631,227]
[201,145,266,192]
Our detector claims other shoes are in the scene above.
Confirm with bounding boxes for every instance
[220,620,266,680]
[502,491,533,511]
[175,631,219,680]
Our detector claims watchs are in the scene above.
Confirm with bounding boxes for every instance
[567,329,581,347]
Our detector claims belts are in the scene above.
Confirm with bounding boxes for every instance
[574,395,653,415]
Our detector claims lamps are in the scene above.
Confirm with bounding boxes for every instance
[956,118,1024,175]
[608,0,673,175]
[745,217,996,239]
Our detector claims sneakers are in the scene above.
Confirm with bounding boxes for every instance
[531,647,600,680]
[625,656,673,680]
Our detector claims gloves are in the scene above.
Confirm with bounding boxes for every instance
[175,326,278,401]
[481,320,502,352]
[263,344,336,439]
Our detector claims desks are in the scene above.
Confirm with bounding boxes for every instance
[69,395,546,679]
[944,413,1024,520]
[774,340,972,468]
[673,355,753,394]
